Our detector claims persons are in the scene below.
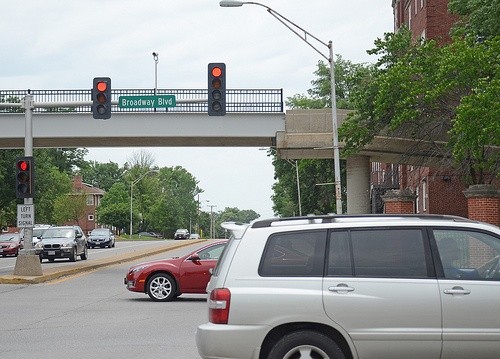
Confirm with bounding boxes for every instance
[438,238,500,279]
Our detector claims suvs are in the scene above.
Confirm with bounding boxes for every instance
[195,212,500,359]
[174,229,189,240]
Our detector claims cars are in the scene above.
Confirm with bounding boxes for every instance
[86,228,115,249]
[147,231,163,239]
[0,223,89,262]
[189,233,201,239]
[123,239,230,302]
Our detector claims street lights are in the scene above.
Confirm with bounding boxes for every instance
[259,146,302,217]
[130,170,159,238]
[197,194,210,234]
[152,52,158,111]
[220,0,342,216]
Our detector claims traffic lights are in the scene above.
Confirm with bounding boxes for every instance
[207,63,226,116]
[93,77,111,119]
[16,156,35,197]
